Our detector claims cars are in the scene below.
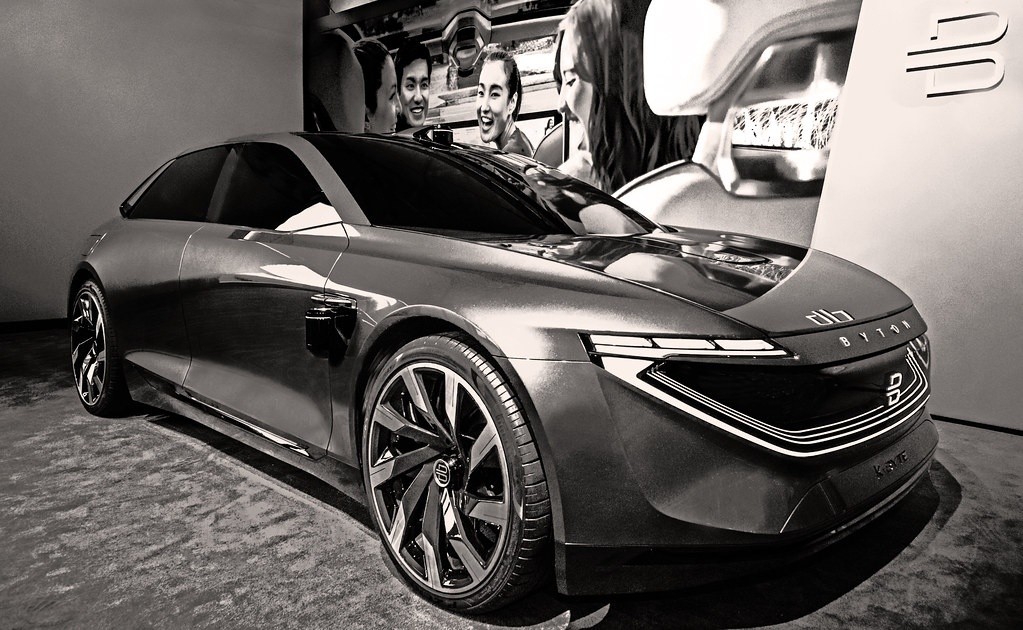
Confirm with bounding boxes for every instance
[66,122,940,618]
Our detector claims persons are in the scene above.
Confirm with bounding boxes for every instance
[394,42,431,133]
[553,0,700,193]
[476,51,534,158]
[353,38,402,134]
